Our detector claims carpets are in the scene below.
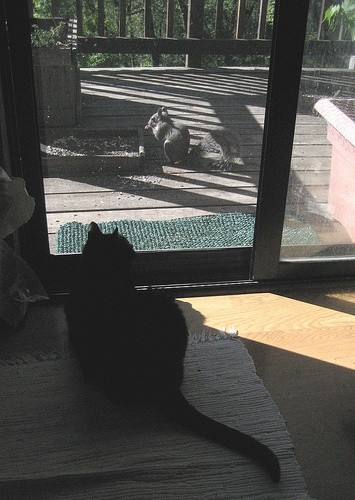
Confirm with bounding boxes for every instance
[57,212,323,254]
[0,327,311,500]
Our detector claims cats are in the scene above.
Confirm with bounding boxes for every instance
[64,222,281,483]
